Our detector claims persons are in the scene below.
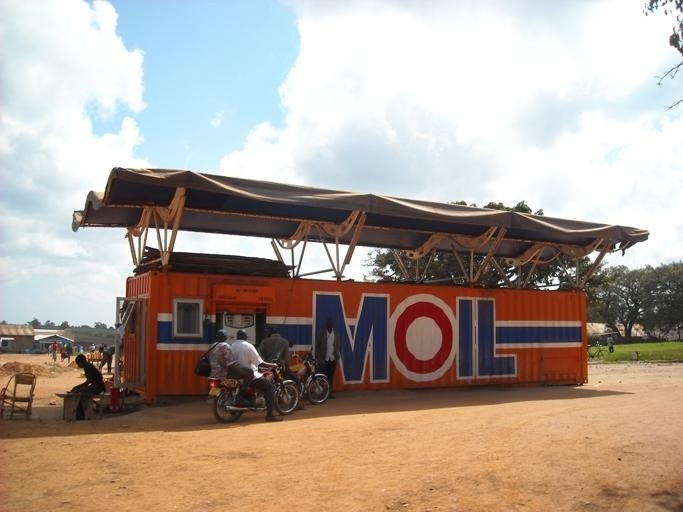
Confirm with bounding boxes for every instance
[606,333,615,353]
[98,342,104,352]
[253,325,306,410]
[98,346,115,372]
[88,343,96,354]
[194,328,255,408]
[65,353,105,421]
[229,328,283,423]
[46,339,84,363]
[313,316,340,399]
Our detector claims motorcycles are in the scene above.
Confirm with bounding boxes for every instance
[277,341,331,405]
[207,359,301,423]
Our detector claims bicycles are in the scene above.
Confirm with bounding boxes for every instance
[586,340,608,362]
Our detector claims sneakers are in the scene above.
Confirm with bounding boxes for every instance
[328,394,336,399]
[239,389,251,401]
[266,415,284,422]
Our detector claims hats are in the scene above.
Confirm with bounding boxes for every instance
[216,329,229,336]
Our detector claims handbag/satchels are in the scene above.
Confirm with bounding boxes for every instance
[193,342,219,377]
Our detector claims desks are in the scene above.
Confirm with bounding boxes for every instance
[55,388,92,423]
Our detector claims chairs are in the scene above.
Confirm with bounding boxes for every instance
[92,372,127,421]
[0,372,36,421]
